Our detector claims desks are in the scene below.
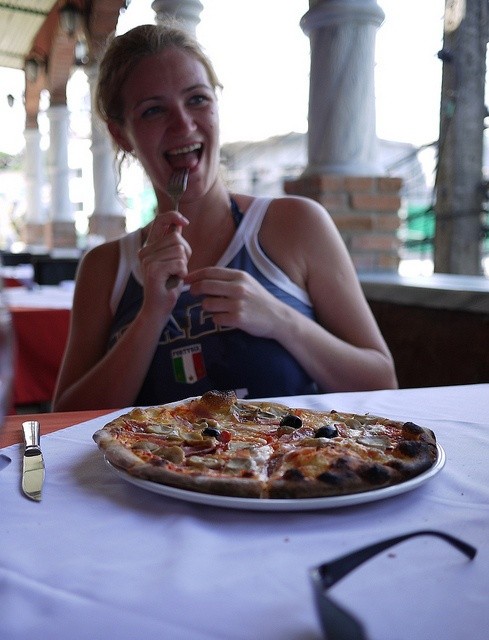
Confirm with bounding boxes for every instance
[3,287,74,404]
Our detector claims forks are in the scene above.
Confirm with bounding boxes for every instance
[165,167,189,291]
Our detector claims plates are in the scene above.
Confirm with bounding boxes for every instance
[107,411,448,514]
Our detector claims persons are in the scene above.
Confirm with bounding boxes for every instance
[48,23,400,416]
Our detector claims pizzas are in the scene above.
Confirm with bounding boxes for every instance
[92,392,438,497]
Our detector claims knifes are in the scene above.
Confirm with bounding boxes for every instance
[19,418,46,503]
